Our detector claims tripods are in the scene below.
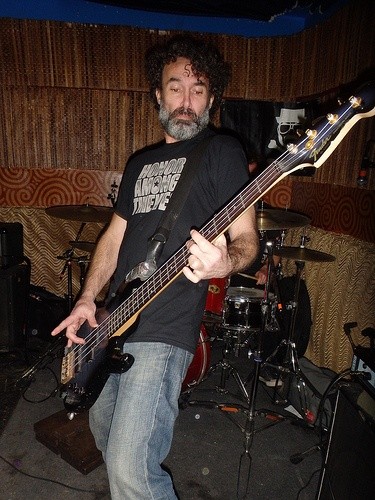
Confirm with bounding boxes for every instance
[201,230,313,431]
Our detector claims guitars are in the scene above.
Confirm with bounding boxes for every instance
[61,91,375,422]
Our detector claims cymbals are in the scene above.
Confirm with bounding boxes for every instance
[254,208,312,232]
[68,240,97,253]
[45,203,116,223]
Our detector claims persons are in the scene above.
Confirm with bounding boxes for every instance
[50,30,259,500]
[226,143,280,329]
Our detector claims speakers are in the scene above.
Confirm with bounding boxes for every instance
[316,379,374,500]
[0,264,28,355]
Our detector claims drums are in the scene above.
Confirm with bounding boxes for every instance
[182,322,211,395]
[273,245,336,263]
[203,276,229,325]
[221,285,276,332]
